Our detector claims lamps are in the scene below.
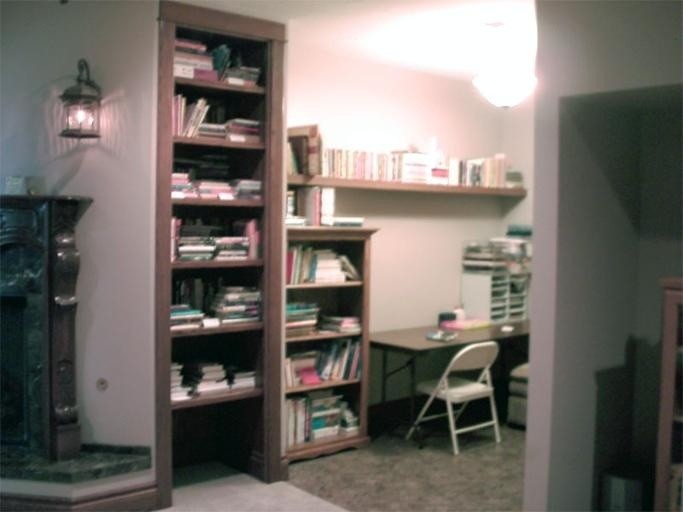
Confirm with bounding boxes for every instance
[470,65,538,107]
[53,56,101,142]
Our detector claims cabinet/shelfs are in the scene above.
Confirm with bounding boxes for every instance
[155,0,287,512]
[461,267,528,323]
[647,275,682,512]
[278,223,379,468]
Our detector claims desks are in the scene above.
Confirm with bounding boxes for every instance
[361,319,528,451]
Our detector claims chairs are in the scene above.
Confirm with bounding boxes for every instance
[404,337,500,455]
